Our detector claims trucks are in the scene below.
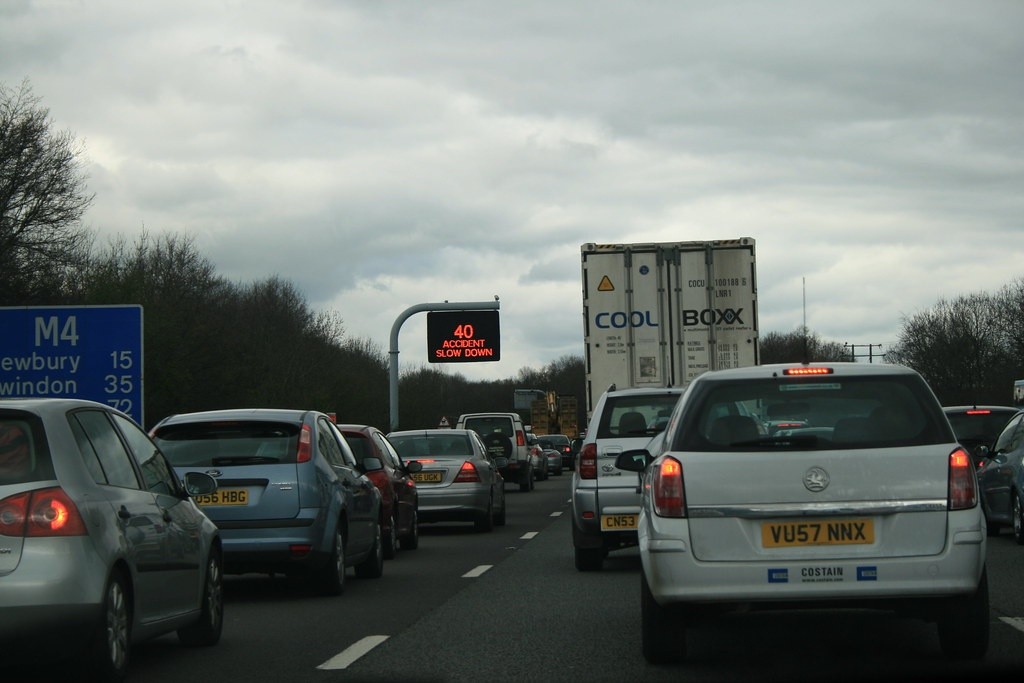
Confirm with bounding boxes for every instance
[574,235,762,435]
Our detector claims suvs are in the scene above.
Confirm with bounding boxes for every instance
[455,413,539,492]
[613,360,994,665]
[568,382,766,572]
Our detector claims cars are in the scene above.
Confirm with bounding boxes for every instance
[384,428,509,534]
[0,395,226,676]
[536,433,571,467]
[336,423,425,559]
[146,405,383,596]
[942,405,1021,470]
[526,432,549,482]
[540,438,564,476]
[764,418,813,438]
[972,408,1024,546]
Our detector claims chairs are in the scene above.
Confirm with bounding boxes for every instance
[619,412,646,436]
[832,417,879,444]
[710,416,759,444]
